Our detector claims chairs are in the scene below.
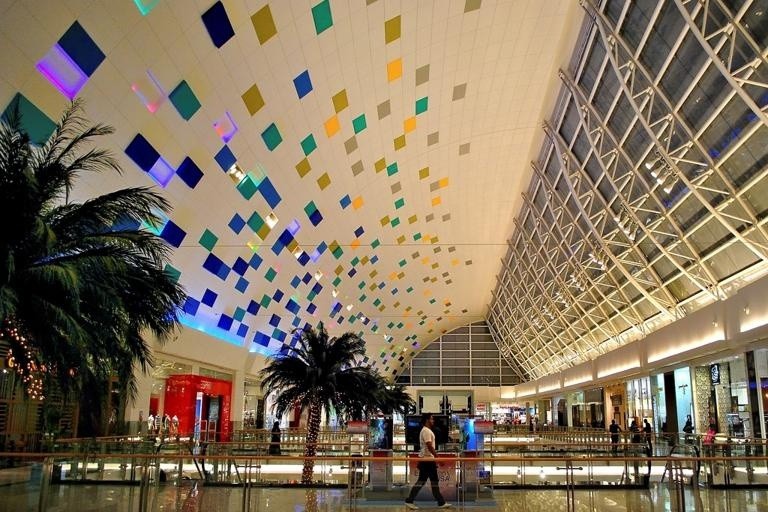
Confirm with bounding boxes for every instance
[438,503,451,508]
[405,503,418,510]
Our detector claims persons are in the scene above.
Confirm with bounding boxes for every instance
[268,421,282,455]
[629,420,641,444]
[643,418,653,451]
[146,413,179,438]
[609,418,621,451]
[404,413,452,511]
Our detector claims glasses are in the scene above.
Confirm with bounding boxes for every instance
[531,117,702,329]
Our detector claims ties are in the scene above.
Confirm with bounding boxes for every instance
[405,415,449,445]
